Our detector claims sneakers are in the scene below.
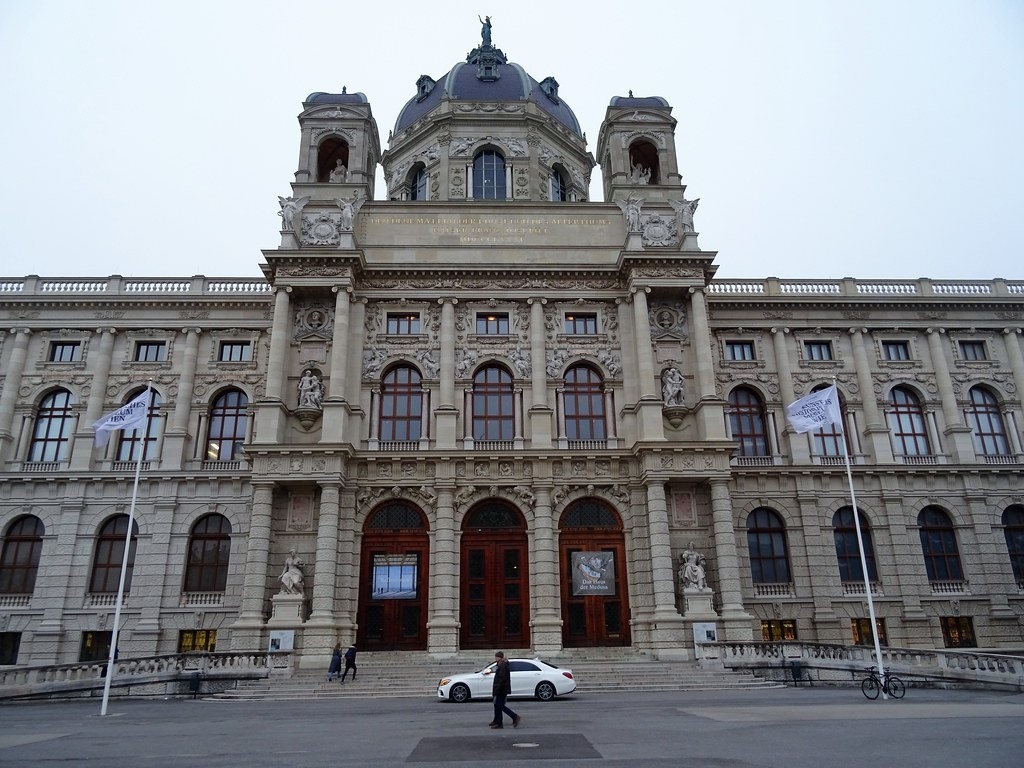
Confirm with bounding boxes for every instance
[489,720,503,729]
[513,716,521,727]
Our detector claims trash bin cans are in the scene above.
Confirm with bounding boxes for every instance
[793,661,801,679]
[189,672,200,691]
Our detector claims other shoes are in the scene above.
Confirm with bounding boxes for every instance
[341,681,344,683]
[352,678,355,680]
[328,678,332,681]
[337,676,341,679]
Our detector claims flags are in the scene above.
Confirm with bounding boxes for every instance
[92,390,149,449]
[786,386,841,434]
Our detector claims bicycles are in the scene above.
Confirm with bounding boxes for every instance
[861,665,907,701]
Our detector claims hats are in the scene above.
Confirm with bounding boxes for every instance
[495,651,504,658]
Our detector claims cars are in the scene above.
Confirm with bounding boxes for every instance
[435,656,578,704]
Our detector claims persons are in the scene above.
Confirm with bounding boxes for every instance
[477,14,492,46]
[329,159,347,183]
[342,197,354,230]
[682,202,695,232]
[278,548,304,598]
[679,541,706,592]
[282,196,297,230]
[298,370,322,409]
[328,642,357,685]
[488,651,522,730]
[662,368,686,407]
[630,155,646,180]
[625,198,640,231]
